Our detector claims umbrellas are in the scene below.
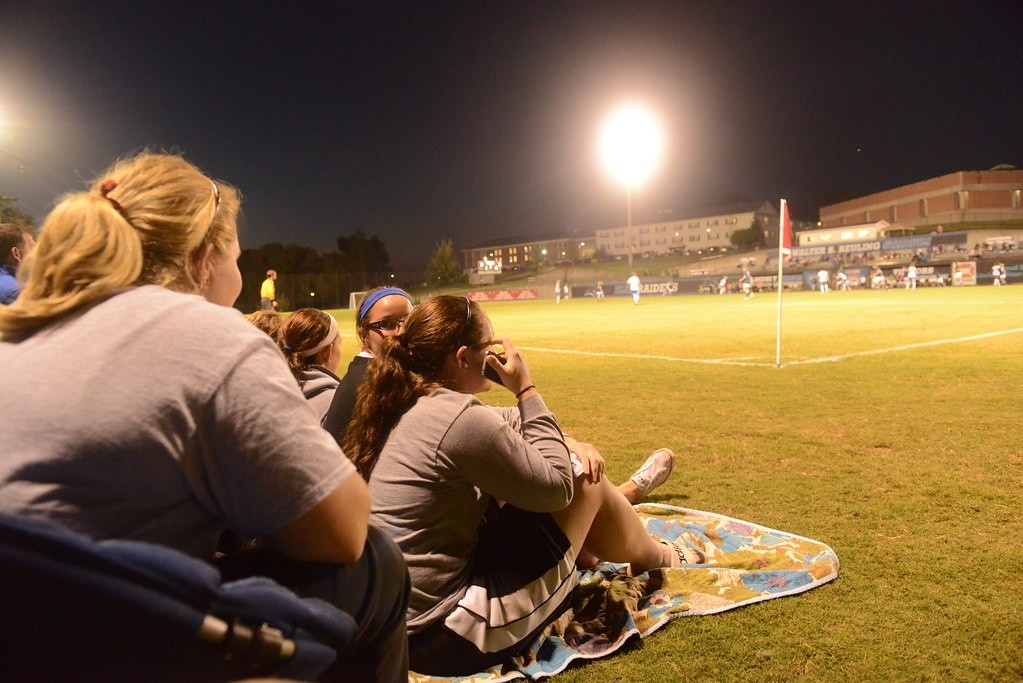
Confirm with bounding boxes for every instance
[782,203,793,249]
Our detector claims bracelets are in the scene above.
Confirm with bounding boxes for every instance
[516,385,536,398]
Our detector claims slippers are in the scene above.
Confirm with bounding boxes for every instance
[576,555,603,573]
[659,541,705,567]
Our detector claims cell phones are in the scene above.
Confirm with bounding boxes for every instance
[481,350,507,387]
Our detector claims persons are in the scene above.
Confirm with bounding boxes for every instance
[563,283,568,300]
[554,280,561,302]
[248,310,283,343]
[0,223,36,305]
[789,250,951,292]
[954,241,1014,260]
[321,285,415,446]
[719,258,770,297]
[260,270,278,311]
[596,280,604,298]
[627,271,641,304]
[992,260,1007,286]
[278,307,342,426]
[344,296,703,677]
[0,147,411,683]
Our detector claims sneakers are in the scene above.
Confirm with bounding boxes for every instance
[630,449,675,499]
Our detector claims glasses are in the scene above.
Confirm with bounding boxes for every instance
[364,318,404,333]
